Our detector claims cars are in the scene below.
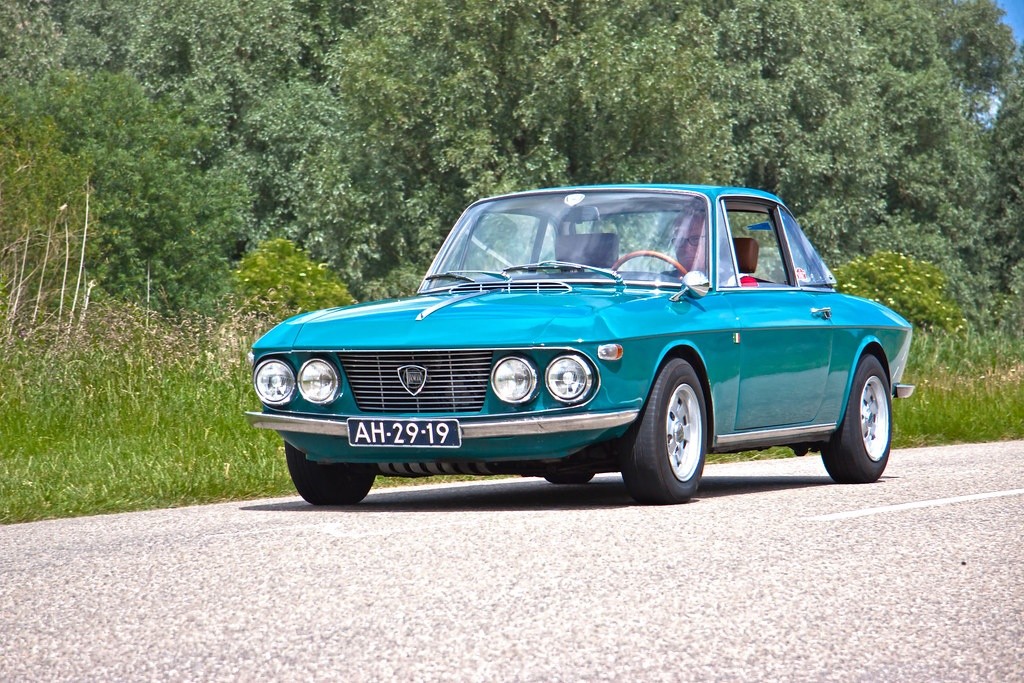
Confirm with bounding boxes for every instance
[244,184,915,509]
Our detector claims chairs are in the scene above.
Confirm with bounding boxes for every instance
[553,233,619,274]
[732,238,759,274]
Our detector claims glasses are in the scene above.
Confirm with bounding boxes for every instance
[671,235,706,248]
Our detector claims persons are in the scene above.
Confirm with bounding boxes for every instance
[633,212,755,284]
